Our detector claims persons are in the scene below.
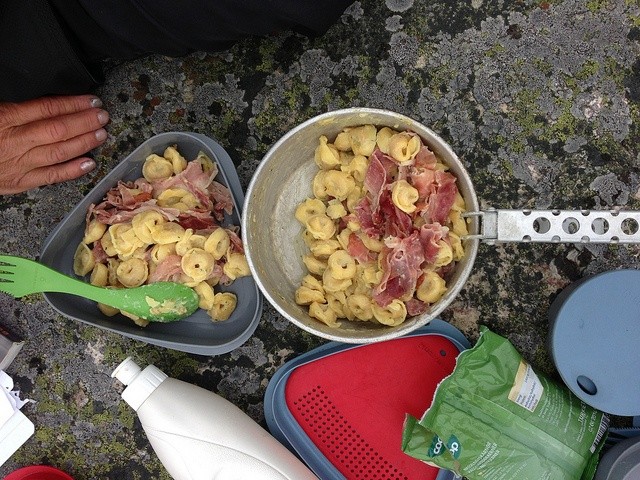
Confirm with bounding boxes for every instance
[0,0,357,197]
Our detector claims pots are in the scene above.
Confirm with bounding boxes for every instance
[241,107,640,343]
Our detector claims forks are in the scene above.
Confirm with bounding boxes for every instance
[0,255,200,324]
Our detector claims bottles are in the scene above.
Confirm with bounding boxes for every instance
[109,353,316,480]
